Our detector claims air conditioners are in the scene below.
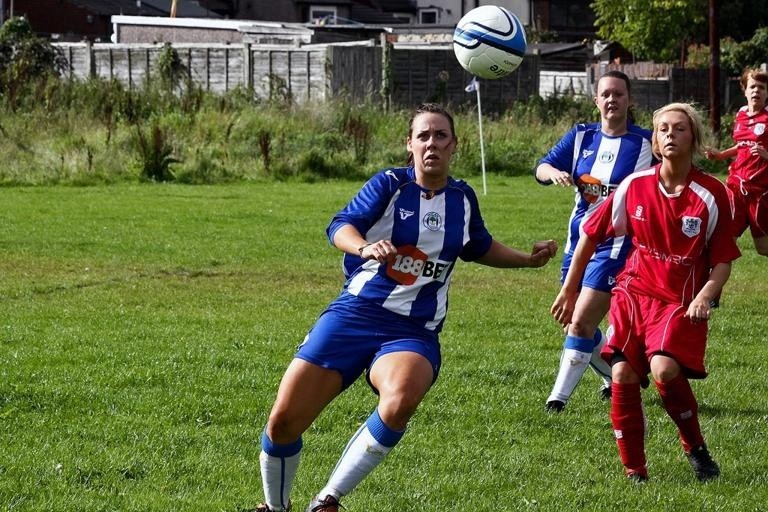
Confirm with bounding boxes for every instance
[309,5,337,25]
[417,8,438,25]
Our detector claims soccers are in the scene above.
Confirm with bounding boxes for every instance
[452,5,528,79]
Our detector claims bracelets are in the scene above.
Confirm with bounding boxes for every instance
[356,243,373,259]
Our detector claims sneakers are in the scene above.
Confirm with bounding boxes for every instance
[253,495,341,512]
[682,441,721,486]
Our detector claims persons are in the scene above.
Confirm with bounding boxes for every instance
[696,70,768,277]
[547,97,744,484]
[529,68,664,412]
[254,100,560,512]
